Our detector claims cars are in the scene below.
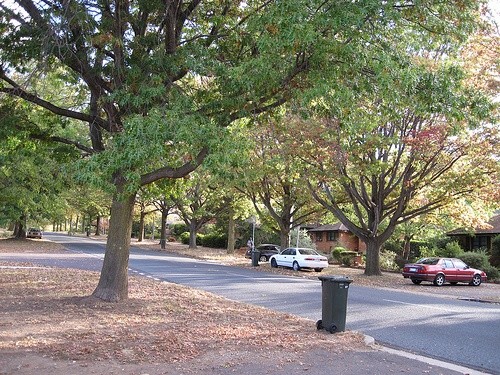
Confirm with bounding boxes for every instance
[26,227,42,239]
[245,244,281,262]
[401,257,487,287]
[269,247,329,272]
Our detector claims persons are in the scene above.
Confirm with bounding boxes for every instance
[245,237,255,259]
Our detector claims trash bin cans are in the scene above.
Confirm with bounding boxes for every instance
[314,275,353,334]
